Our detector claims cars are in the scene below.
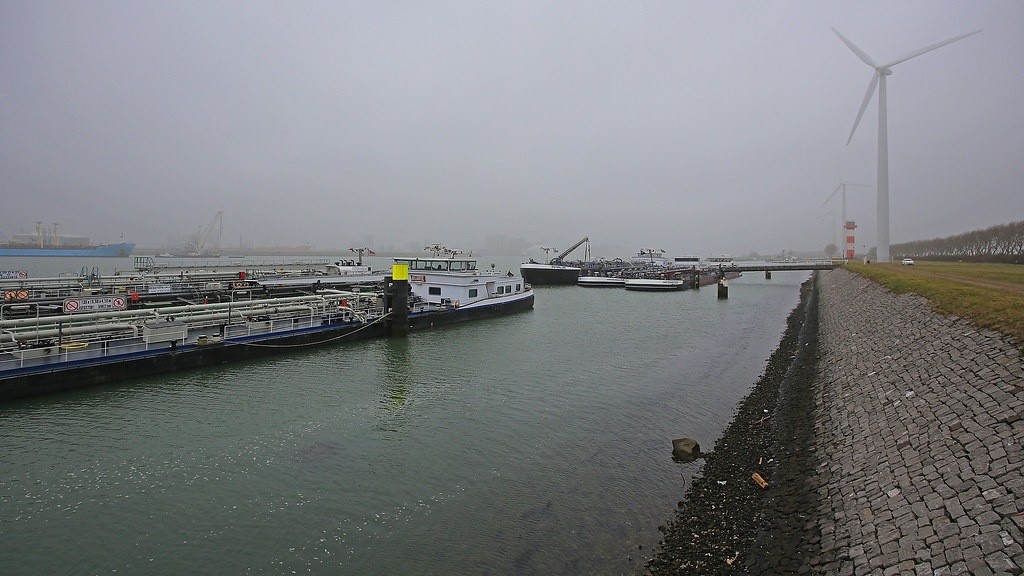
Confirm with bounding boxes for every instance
[901,258,915,265]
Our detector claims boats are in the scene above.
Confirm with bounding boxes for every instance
[520,234,592,287]
